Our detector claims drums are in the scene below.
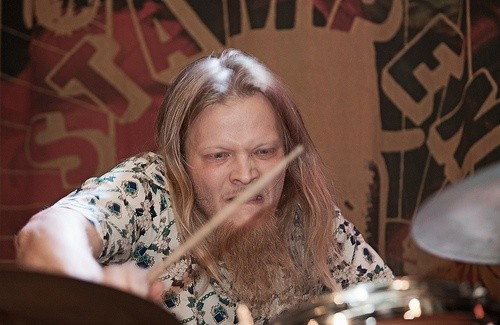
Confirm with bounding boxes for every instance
[262,272,500,325]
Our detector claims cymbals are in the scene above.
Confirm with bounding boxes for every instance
[0,261,182,325]
[411,162,500,265]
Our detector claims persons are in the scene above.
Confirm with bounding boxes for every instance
[14,45,404,325]
